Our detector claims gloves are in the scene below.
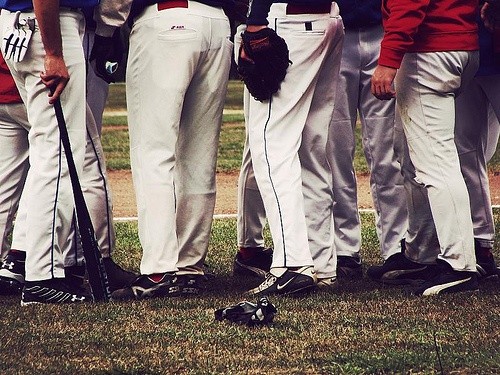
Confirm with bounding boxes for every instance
[1,12,33,64]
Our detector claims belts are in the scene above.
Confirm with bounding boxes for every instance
[283,1,333,16]
[154,0,186,11]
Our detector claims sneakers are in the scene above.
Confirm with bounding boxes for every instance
[232,246,273,282]
[335,255,363,279]
[474,252,496,276]
[110,273,179,302]
[84,257,140,290]
[19,279,92,306]
[179,265,217,295]
[0,252,25,287]
[415,271,474,297]
[316,276,337,296]
[242,265,317,300]
[366,253,437,286]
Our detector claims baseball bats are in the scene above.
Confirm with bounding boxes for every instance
[49,86,113,303]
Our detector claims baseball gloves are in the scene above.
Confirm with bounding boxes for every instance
[90,35,128,82]
[239,28,292,103]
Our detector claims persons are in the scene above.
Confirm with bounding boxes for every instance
[366,0,480,297]
[237,0,345,299]
[57,8,110,285]
[0,0,101,308]
[234,81,274,278]
[112,0,234,300]
[455,0,500,281]
[0,48,141,297]
[326,0,410,282]
